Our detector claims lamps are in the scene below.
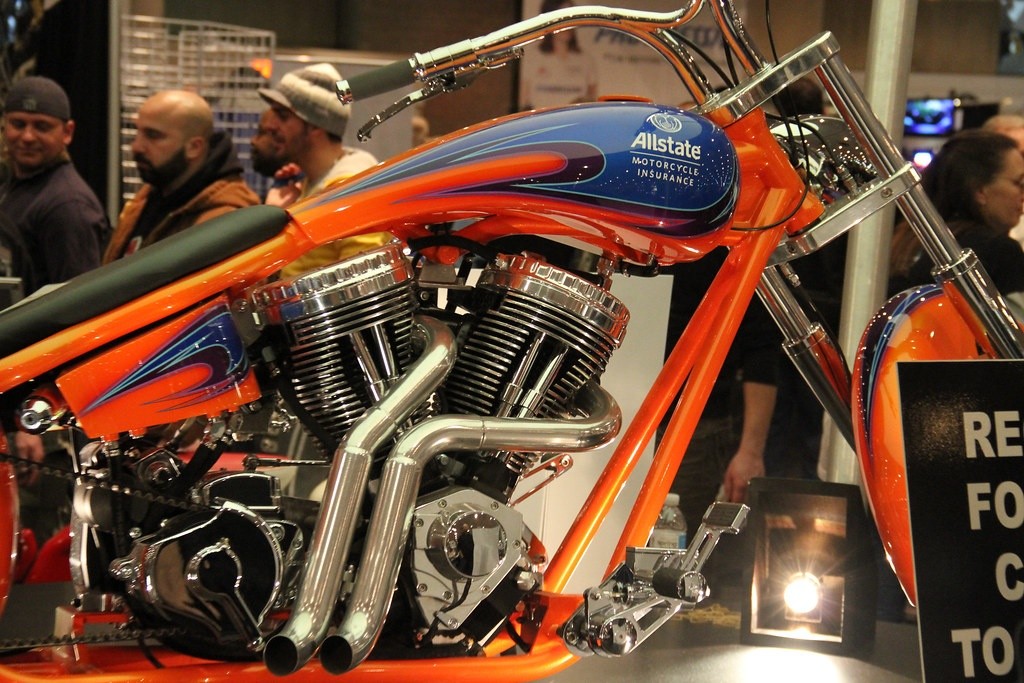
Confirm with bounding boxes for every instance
[739,476,880,656]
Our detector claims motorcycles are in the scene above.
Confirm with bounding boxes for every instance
[0,1,1023,683]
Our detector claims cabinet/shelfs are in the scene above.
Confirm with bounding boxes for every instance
[118,13,276,231]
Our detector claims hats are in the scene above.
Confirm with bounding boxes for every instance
[257,63,352,136]
[4,76,70,120]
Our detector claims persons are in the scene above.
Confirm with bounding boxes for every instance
[650,84,782,613]
[0,76,109,544]
[103,89,277,454]
[263,61,393,279]
[766,71,848,479]
[250,107,293,177]
[983,112,1024,245]
[889,129,1024,297]
